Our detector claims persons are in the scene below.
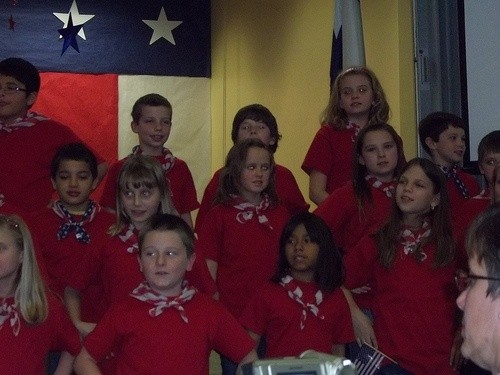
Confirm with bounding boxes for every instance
[194,103,309,231]
[28,142,122,302]
[337,157,473,375]
[491,163,500,205]
[301,67,391,207]
[0,213,83,375]
[418,111,483,213]
[312,122,409,325]
[99,93,201,232]
[454,131,500,257]
[455,200,500,375]
[196,139,297,373]
[237,211,355,359]
[73,213,260,375]
[0,57,110,218]
[63,154,221,338]
